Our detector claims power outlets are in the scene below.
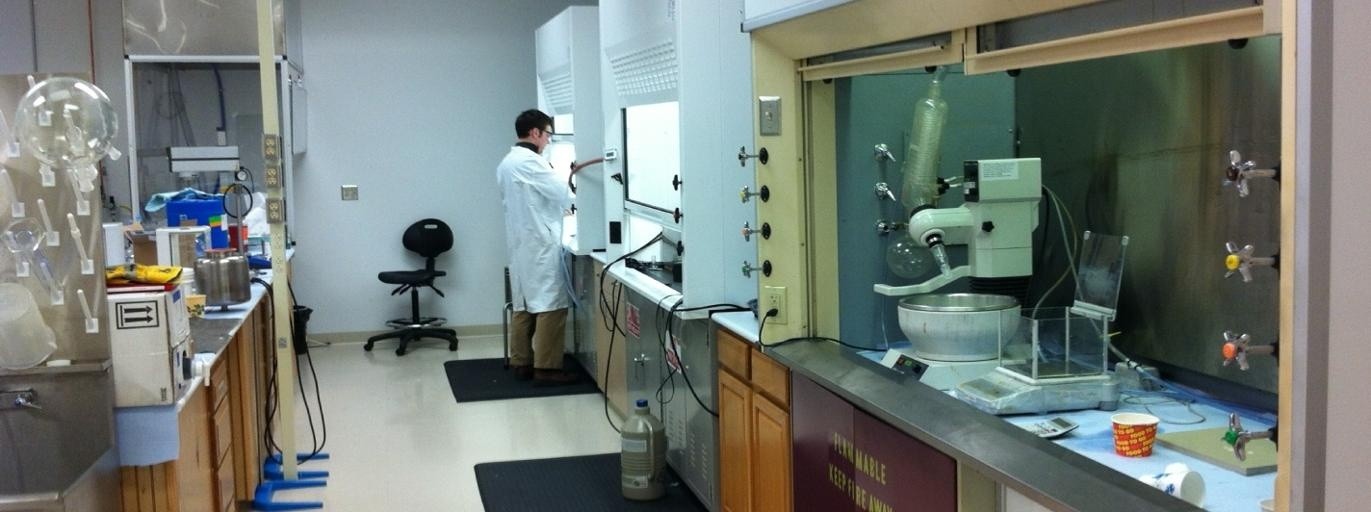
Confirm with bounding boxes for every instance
[764,285,787,325]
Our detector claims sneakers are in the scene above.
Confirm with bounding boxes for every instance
[515,367,571,385]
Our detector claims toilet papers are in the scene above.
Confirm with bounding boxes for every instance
[102,222,128,268]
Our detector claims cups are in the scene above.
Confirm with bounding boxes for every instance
[185,293,207,318]
[1139,462,1206,509]
[1111,412,1160,457]
[0,281,59,372]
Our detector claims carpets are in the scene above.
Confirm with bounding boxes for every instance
[475,452,710,512]
[444,354,603,403]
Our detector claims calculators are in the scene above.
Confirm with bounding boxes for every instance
[1025,416,1080,438]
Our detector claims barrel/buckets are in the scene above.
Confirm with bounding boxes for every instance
[619,399,666,500]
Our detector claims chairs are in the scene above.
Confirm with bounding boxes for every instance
[363,218,458,357]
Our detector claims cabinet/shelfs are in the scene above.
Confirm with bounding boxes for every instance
[120,311,261,512]
[711,327,793,512]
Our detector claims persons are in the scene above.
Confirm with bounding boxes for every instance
[496,109,579,384]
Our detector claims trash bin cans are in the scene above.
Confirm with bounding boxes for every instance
[294,305,313,354]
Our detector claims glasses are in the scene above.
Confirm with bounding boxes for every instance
[541,129,553,139]
